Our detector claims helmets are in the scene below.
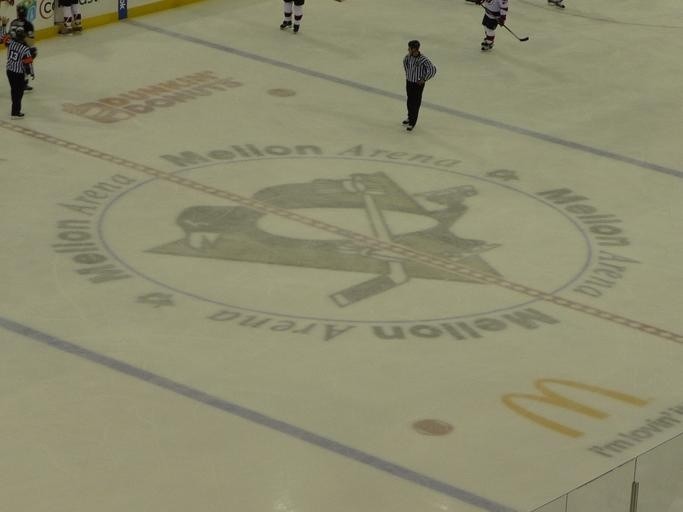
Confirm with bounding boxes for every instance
[16,5,28,18]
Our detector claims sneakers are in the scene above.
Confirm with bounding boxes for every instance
[293,23,300,32]
[406,124,415,133]
[23,85,34,91]
[11,112,25,117]
[279,20,293,28]
[480,41,495,52]
[401,118,410,127]
[58,24,83,35]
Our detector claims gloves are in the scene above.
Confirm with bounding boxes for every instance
[476,0,482,5]
[498,19,505,26]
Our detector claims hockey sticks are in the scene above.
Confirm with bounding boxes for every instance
[479,2,529,42]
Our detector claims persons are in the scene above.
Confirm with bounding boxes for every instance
[5,27,34,118]
[402,39,436,131]
[280,0,303,31]
[56,1,82,34]
[8,5,35,91]
[473,0,508,50]
